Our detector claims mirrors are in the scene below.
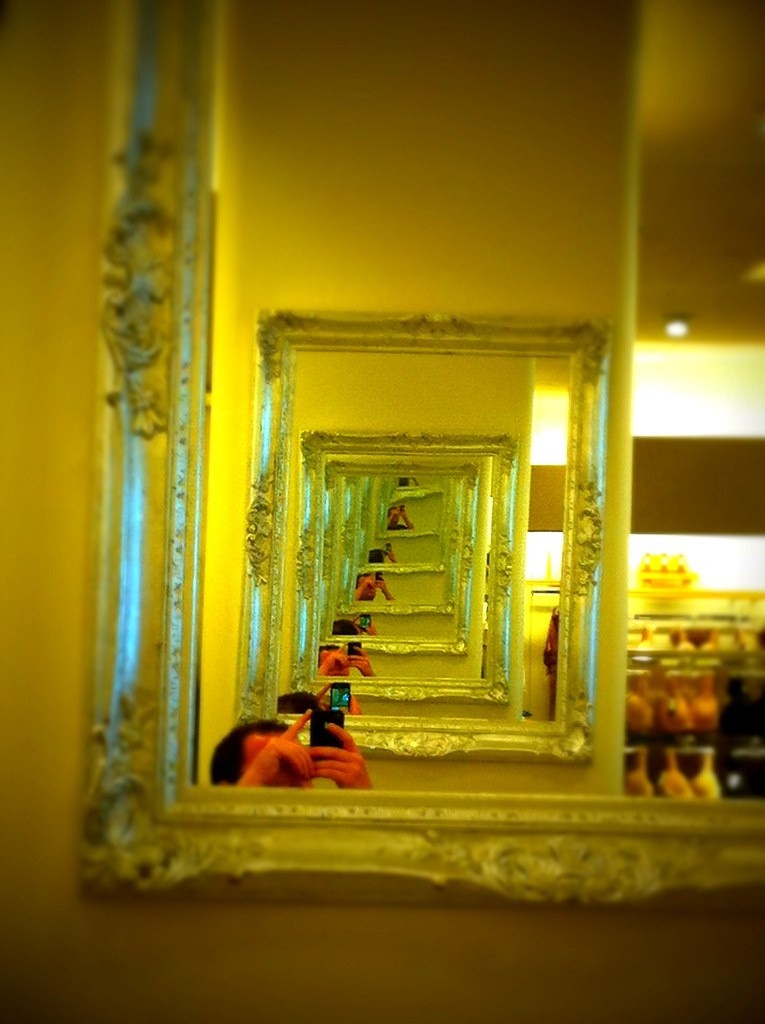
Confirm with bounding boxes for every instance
[65,2,765,904]
[233,300,604,765]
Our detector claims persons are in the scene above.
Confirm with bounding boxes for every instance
[369,543,397,563]
[277,682,364,715]
[318,642,377,677]
[398,477,418,487]
[332,613,379,636]
[355,571,396,601]
[210,710,375,790]
[386,504,414,531]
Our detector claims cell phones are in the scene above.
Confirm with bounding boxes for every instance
[310,506,406,762]
